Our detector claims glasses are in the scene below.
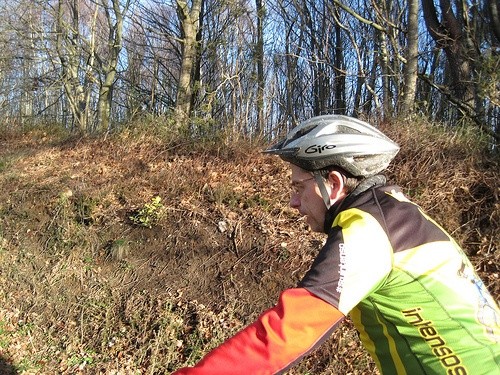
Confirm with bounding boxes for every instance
[290,174,319,194]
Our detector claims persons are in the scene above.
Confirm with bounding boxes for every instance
[172,114,500,375]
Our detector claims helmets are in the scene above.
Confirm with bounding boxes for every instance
[262,113,401,178]
